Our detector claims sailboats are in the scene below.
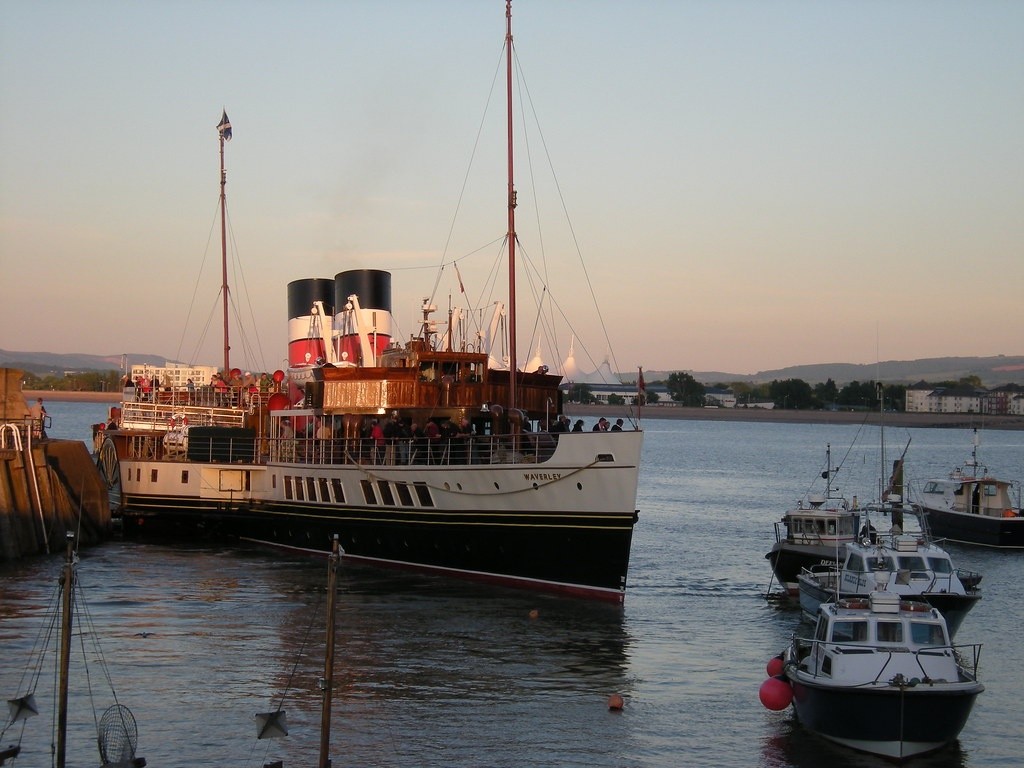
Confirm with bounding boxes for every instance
[0,528,151,768]
[239,536,405,768]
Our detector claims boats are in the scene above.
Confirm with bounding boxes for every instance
[762,323,1024,759]
[90,0,649,606]
[0,365,112,563]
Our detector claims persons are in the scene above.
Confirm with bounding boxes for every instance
[859,519,876,544]
[161,372,172,405]
[210,372,272,408]
[31,398,46,419]
[281,414,625,465]
[186,379,194,391]
[121,375,160,401]
[106,418,117,430]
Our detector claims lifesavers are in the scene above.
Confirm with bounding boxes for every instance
[839,597,870,609]
[170,414,188,431]
[981,477,995,480]
[901,600,932,612]
[961,478,975,481]
